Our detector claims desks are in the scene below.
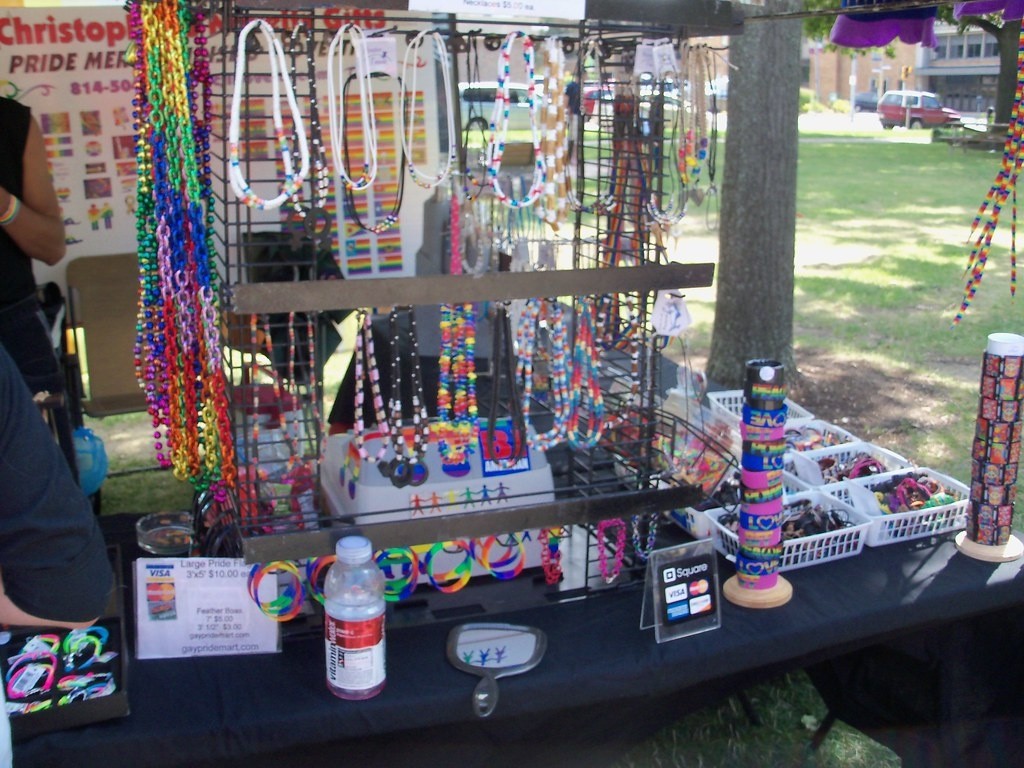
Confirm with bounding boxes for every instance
[0,304,1023,768]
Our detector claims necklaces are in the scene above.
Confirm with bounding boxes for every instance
[131,0,718,496]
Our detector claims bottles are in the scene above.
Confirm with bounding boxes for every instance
[322,535,389,702]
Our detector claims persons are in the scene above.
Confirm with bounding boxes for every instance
[0,95,76,484]
[0,341,111,630]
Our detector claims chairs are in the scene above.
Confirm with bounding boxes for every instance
[48,250,168,514]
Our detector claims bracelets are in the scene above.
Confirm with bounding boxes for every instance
[249,512,664,623]
[6,625,115,715]
[0,195,20,225]
[736,384,789,589]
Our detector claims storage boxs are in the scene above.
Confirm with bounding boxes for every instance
[731,420,861,458]
[648,469,810,537]
[707,388,815,424]
[703,490,876,571]
[823,467,970,549]
[782,442,916,488]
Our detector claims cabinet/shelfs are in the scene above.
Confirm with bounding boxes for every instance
[131,0,724,632]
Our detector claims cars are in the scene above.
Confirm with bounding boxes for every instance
[459,80,557,132]
[583,78,617,117]
[852,91,883,113]
[877,89,962,130]
[589,89,713,131]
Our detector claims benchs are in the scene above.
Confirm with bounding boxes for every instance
[942,119,1009,147]
